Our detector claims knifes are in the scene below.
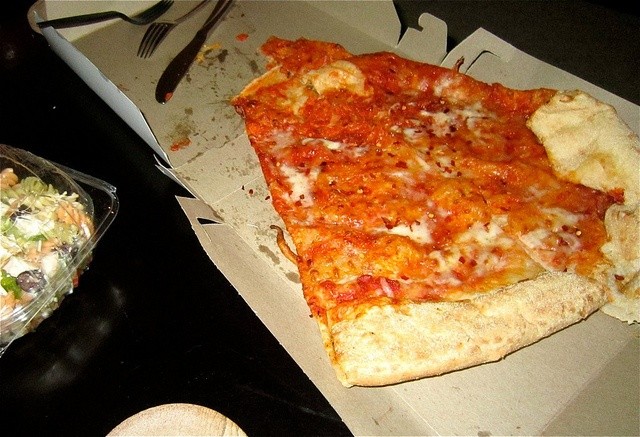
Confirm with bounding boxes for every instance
[156,0,235,105]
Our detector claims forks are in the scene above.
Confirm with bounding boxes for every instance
[137,0,212,58]
[37,0,174,28]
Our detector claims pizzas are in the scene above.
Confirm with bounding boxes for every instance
[230,34,636,389]
